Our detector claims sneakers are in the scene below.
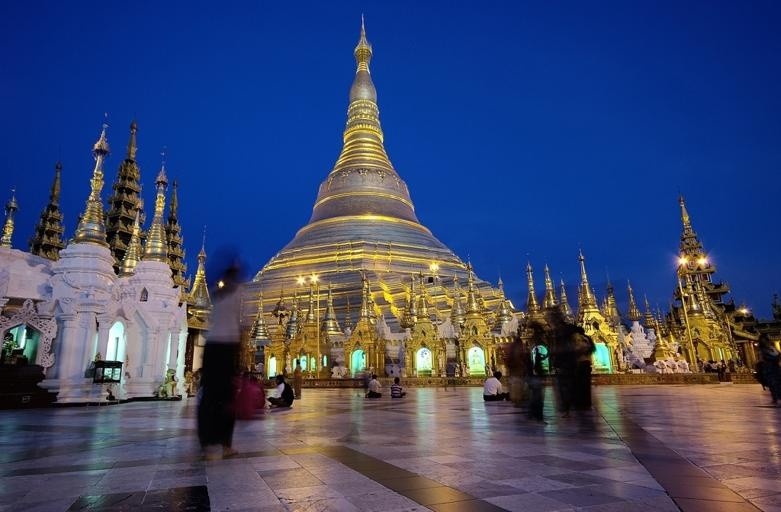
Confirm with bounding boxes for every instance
[223,451,237,458]
[199,453,213,461]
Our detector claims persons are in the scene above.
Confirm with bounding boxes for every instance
[184,359,303,420]
[507,307,596,424]
[390,377,406,398]
[364,374,382,399]
[195,259,253,459]
[697,331,780,406]
[362,370,369,394]
[482,371,509,401]
[446,360,493,392]
